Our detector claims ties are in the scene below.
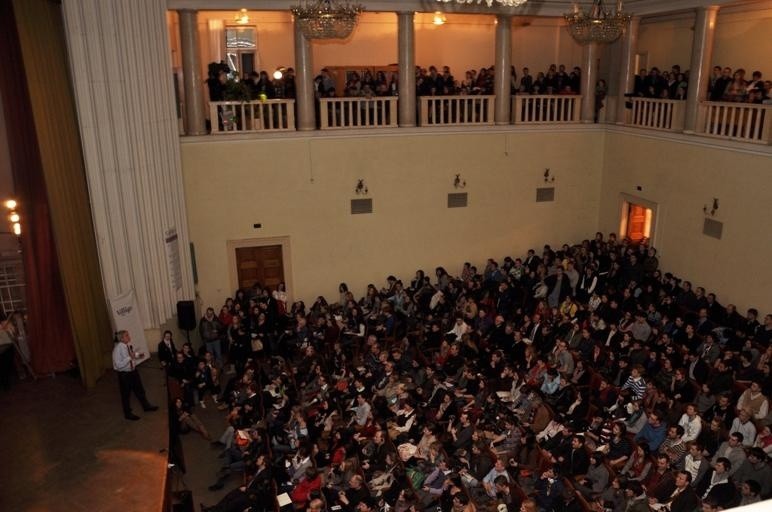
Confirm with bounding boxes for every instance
[127,345,133,369]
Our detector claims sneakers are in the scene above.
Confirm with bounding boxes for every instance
[212,395,218,403]
[200,401,206,409]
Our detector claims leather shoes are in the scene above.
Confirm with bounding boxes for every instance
[210,440,227,449]
[218,402,228,410]
[199,503,209,511]
[208,484,225,491]
[143,405,158,412]
[215,467,231,477]
[125,413,140,421]
[218,448,230,459]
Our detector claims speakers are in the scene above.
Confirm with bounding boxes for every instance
[176,300,197,330]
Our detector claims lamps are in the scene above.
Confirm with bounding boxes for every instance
[290,0,367,40]
[235,8,248,25]
[544,168,555,185]
[2,197,22,244]
[703,196,719,216]
[432,10,447,25]
[273,66,283,79]
[453,174,466,189]
[560,0,637,40]
[355,178,368,195]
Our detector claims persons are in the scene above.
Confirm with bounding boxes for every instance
[158,228,772,512]
[112,329,160,422]
[199,61,771,142]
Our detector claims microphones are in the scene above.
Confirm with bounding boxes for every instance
[129,344,134,352]
